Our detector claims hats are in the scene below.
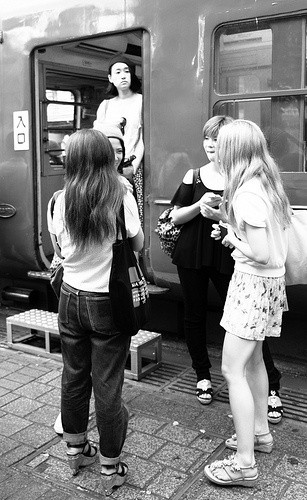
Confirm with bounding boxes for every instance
[108,52,136,74]
[93,120,125,148]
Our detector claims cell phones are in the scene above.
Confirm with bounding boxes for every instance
[218,225,236,239]
[210,195,222,201]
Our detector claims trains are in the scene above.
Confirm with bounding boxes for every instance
[1,0,307,297]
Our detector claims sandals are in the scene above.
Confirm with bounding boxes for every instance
[267,390,284,424]
[225,432,273,453]
[204,453,258,488]
[196,374,213,405]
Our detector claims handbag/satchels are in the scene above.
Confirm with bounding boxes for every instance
[109,238,149,336]
[284,209,307,286]
[154,208,181,257]
[50,265,63,299]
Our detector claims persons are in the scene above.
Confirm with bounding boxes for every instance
[54,58,144,435]
[169,115,284,425]
[47,128,145,495]
[199,119,293,487]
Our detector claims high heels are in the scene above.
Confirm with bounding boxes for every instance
[66,439,98,474]
[100,457,128,496]
